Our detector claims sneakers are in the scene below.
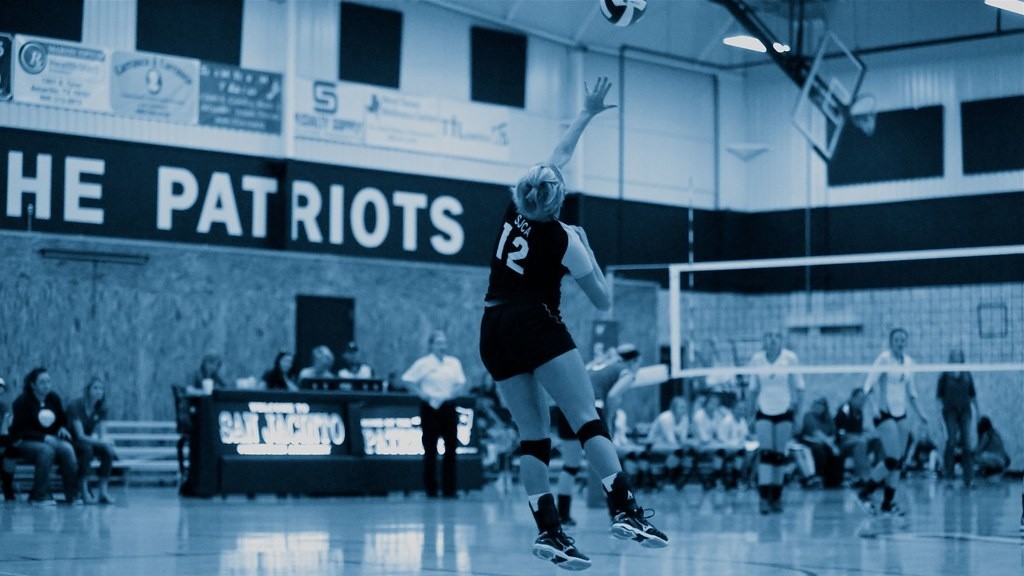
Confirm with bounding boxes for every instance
[610,507,668,548]
[532,530,592,571]
[881,502,904,516]
[857,490,877,516]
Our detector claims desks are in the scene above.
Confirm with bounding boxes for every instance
[186,392,486,500]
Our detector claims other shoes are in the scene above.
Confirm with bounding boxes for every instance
[760,498,783,515]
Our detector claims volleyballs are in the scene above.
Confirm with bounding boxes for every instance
[599,0,649,28]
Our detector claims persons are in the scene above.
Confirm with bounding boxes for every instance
[470,328,1011,517]
[402,329,467,499]
[479,76,668,571]
[0,367,119,507]
[172,342,377,498]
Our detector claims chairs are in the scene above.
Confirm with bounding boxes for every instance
[171,384,188,469]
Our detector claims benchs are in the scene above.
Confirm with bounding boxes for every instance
[0,419,183,493]
